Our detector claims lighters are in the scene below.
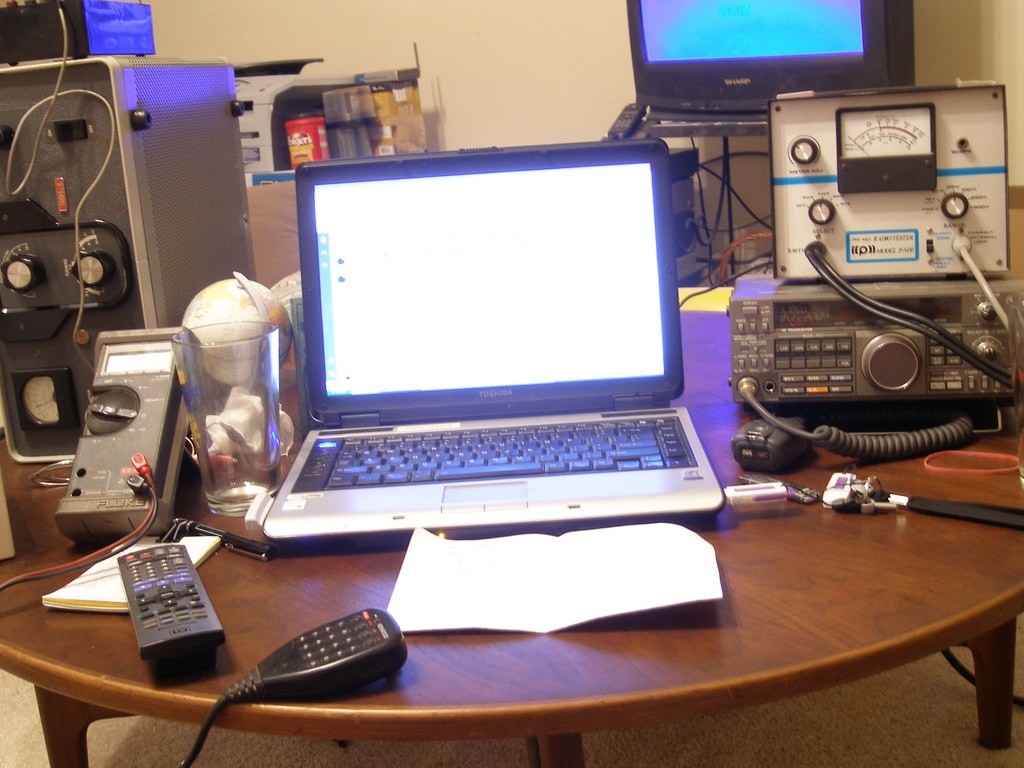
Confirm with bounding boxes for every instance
[738,472,820,505]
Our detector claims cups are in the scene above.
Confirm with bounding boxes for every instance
[172,321,280,516]
[284,101,330,170]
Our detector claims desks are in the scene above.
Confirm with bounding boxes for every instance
[650,121,766,286]
[0,311,1024,768]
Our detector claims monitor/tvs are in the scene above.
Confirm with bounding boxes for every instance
[627,0,915,122]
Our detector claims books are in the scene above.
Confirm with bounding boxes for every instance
[42,535,222,612]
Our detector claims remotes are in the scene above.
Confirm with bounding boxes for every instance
[608,103,646,140]
[118,543,225,661]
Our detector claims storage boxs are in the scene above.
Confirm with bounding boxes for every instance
[322,84,377,125]
[327,125,374,159]
[368,114,429,156]
[370,77,422,127]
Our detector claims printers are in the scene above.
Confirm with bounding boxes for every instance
[234,58,353,172]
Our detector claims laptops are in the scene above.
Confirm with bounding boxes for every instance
[261,137,727,553]
